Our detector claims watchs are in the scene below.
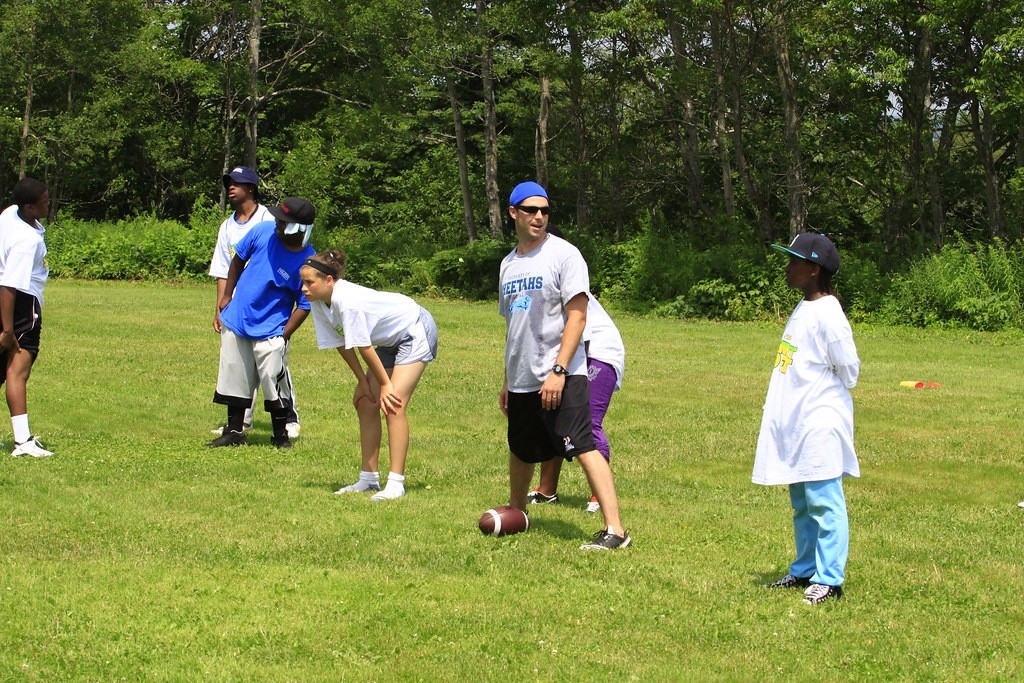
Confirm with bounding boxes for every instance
[552,364,569,375]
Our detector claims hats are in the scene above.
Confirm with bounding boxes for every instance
[221,166,259,193]
[770,232,840,276]
[509,182,549,208]
[267,196,316,224]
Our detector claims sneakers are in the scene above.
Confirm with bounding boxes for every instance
[212,424,247,448]
[579,528,634,552]
[212,421,254,434]
[10,434,54,459]
[271,427,293,450]
[371,485,406,502]
[803,582,845,606]
[286,421,301,438]
[584,493,601,514]
[333,482,381,494]
[765,574,813,589]
[526,486,560,503]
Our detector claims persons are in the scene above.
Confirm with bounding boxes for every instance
[209,167,275,434]
[300,250,439,501]
[0,177,53,457]
[499,183,632,553]
[752,234,860,603]
[527,292,626,512]
[207,198,316,448]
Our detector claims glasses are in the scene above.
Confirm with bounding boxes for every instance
[514,204,550,215]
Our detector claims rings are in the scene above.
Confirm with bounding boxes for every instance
[552,396,557,398]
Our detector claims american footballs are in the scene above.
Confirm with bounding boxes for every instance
[477,505,531,538]
